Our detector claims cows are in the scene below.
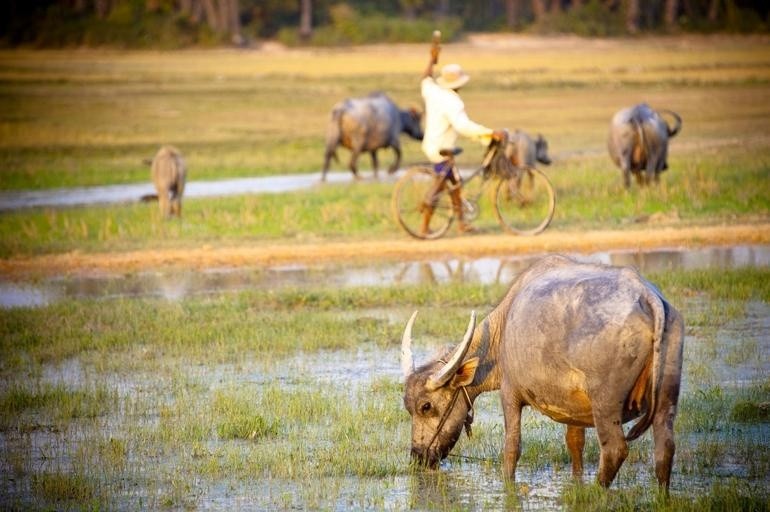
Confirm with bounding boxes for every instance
[141,144,187,218]
[606,102,683,189]
[500,128,553,205]
[398,252,686,505]
[320,92,424,182]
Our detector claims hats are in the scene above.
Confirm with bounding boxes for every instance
[437,64,470,90]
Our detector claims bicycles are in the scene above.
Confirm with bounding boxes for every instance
[392,132,557,240]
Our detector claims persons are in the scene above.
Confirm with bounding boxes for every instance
[418,44,507,240]
